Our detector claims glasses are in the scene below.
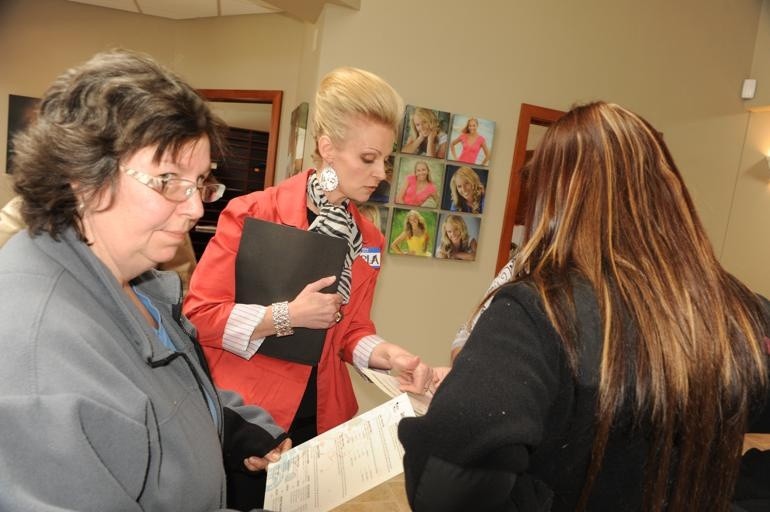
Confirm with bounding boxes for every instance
[117,164,226,203]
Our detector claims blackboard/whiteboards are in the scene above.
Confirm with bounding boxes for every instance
[6,94,42,174]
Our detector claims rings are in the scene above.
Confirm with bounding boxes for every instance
[336,312,342,322]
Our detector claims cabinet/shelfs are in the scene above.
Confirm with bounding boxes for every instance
[190,125,269,263]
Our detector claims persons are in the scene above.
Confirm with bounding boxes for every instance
[2,196,198,299]
[1,49,291,511]
[398,103,770,512]
[390,107,490,260]
[183,69,433,396]
